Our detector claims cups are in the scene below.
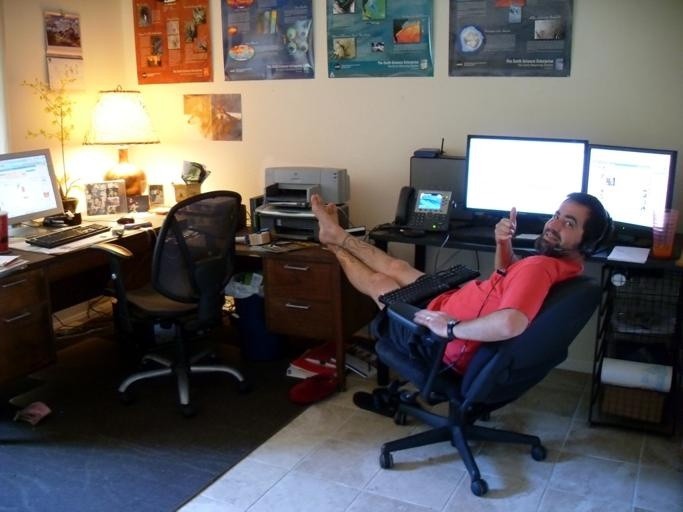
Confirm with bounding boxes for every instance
[650,207,678,259]
[0,211,10,256]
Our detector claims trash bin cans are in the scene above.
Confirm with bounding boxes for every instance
[227,288,265,361]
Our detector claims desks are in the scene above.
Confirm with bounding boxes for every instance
[0,205,367,419]
[370,212,683,386]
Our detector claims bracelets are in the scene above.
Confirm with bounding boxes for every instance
[446,316,460,340]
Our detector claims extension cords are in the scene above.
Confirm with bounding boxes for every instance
[345,343,378,363]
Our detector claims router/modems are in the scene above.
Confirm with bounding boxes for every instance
[414,138,444,158]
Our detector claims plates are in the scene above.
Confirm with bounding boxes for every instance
[227,45,255,63]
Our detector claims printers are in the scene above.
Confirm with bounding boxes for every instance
[250,166,350,243]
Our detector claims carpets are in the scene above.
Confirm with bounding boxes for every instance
[0,332,346,511]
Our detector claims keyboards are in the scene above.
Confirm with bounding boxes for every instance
[378,263,481,305]
[25,223,112,248]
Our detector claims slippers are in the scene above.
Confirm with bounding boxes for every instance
[353,388,400,418]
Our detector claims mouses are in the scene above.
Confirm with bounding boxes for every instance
[116,216,135,225]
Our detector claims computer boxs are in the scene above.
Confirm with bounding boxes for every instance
[410,156,474,221]
[52,295,113,336]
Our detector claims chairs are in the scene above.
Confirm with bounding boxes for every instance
[89,189,252,418]
[369,274,604,498]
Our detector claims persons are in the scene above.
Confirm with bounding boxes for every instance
[86,184,164,216]
[311,192,614,393]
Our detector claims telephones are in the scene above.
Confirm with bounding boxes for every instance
[395,186,454,232]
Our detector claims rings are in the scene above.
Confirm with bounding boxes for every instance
[426,314,431,320]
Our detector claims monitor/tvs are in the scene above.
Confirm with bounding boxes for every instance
[0,148,64,238]
[584,144,678,244]
[462,135,589,234]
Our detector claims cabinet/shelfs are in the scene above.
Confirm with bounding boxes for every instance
[586,261,682,441]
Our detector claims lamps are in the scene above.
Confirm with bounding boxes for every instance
[79,86,162,196]
